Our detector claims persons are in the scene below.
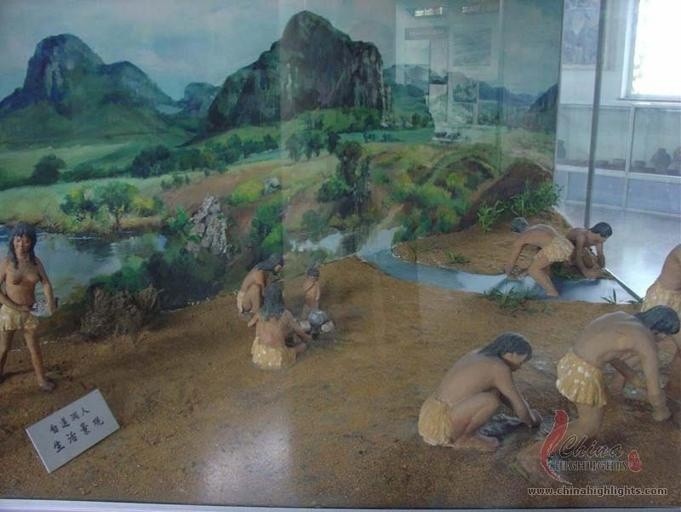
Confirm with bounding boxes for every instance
[235,252,323,371]
[417,331,545,452]
[516,304,680,478]
[0,222,56,393]
[563,221,614,281]
[506,216,577,298]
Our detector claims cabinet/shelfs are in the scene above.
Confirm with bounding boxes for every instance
[544,101,681,218]
